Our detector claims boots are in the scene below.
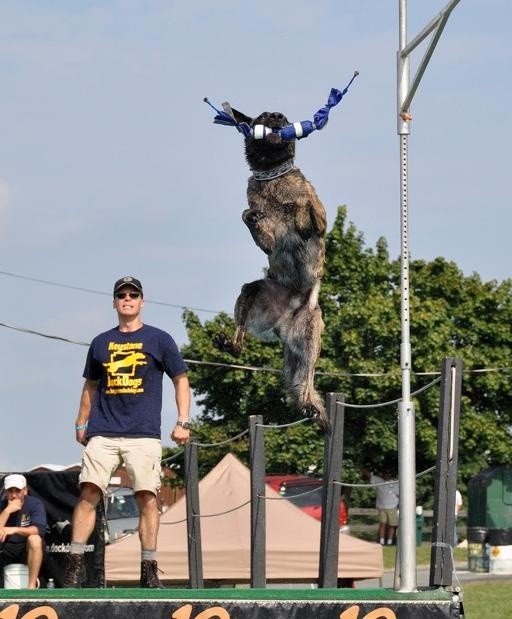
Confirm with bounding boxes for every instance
[140,561,165,588]
[64,554,87,588]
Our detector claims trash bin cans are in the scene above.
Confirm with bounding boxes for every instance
[466,526,512,575]
[415,513,425,546]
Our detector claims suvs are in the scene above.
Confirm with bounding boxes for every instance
[260,470,352,545]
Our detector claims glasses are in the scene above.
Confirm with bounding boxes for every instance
[116,292,140,298]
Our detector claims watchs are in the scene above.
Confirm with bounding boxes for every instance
[175,419,191,430]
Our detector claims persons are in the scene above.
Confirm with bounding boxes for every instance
[360,461,400,546]
[0,471,50,590]
[61,274,194,591]
[455,488,464,547]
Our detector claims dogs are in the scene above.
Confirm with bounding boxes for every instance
[211,108,332,440]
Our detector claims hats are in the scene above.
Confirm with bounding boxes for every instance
[5,474,27,489]
[114,277,143,300]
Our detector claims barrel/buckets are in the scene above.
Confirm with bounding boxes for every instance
[3,564,40,590]
[485,527,512,575]
[466,525,487,573]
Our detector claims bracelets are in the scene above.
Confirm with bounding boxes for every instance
[74,424,87,430]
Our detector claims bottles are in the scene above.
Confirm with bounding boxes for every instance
[47,579,55,589]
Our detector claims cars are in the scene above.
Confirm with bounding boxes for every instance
[101,485,146,545]
